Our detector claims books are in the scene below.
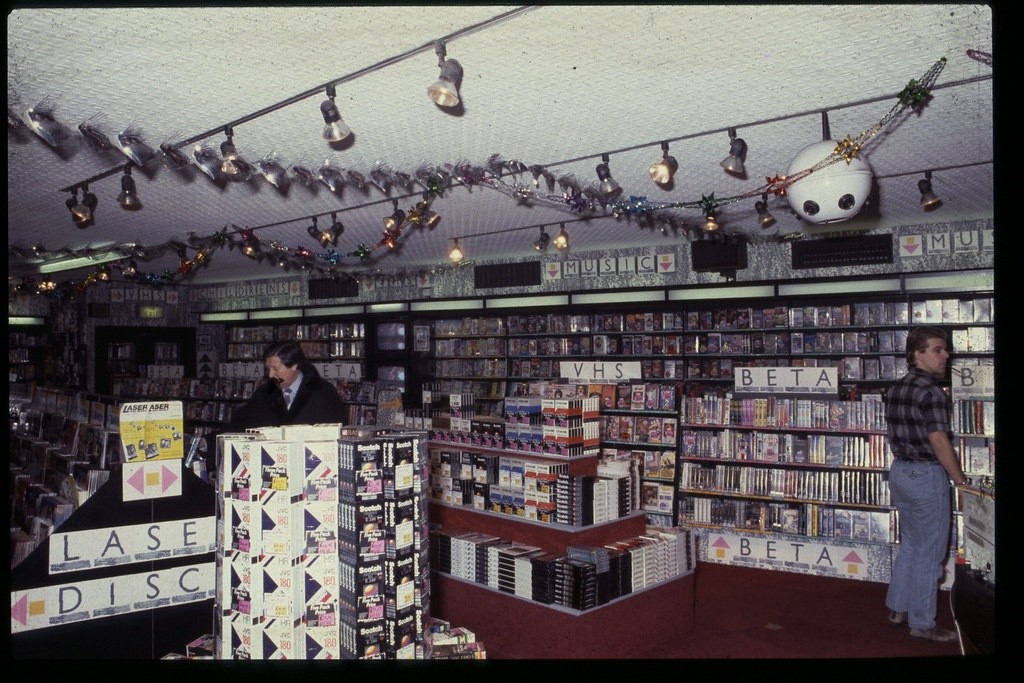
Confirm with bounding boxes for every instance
[7,388,121,571]
[375,298,1000,608]
[103,321,378,459]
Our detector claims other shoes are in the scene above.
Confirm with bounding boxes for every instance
[910,627,958,642]
[889,610,908,625]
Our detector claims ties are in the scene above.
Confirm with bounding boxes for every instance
[284,389,292,406]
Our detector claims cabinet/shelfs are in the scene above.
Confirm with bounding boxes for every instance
[11,388,217,635]
[200,296,994,417]
[392,399,697,616]
[159,424,488,659]
[111,378,405,436]
[679,386,956,592]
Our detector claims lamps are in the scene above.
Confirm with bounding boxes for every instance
[755,195,775,224]
[241,229,258,256]
[596,153,619,195]
[66,187,93,229]
[383,199,405,231]
[918,171,944,206]
[177,249,189,267]
[98,266,111,281]
[115,165,139,205]
[426,40,463,107]
[552,223,568,250]
[649,142,677,185]
[220,127,239,175]
[323,212,344,243]
[720,128,745,173]
[320,83,350,141]
[786,111,873,225]
[416,193,438,225]
[127,260,136,273]
[308,216,326,244]
[704,212,719,231]
[449,238,462,262]
[71,183,96,220]
[532,226,549,253]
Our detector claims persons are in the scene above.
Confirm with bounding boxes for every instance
[555,390,562,399]
[884,326,969,643]
[576,385,584,396]
[188,339,349,487]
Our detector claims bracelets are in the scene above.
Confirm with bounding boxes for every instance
[957,478,968,485]
